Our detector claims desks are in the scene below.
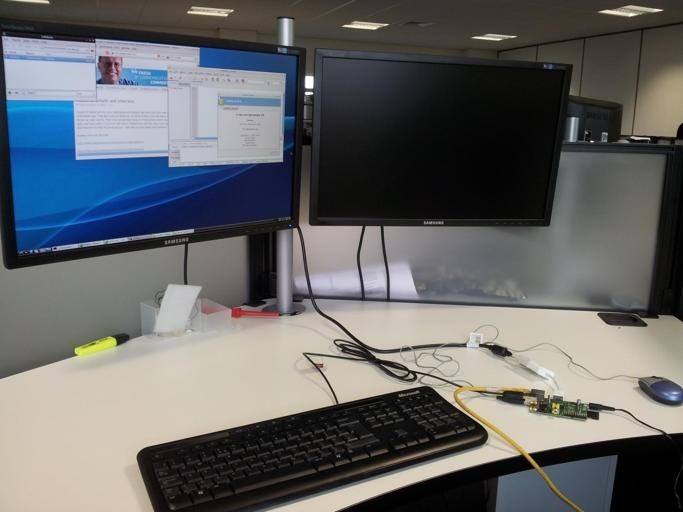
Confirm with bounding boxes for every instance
[1,296,683,512]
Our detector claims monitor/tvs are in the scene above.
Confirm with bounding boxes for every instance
[0,15,308,270]
[567,94,624,143]
[309,48,573,230]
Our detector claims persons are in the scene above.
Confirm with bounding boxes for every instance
[96,55,138,86]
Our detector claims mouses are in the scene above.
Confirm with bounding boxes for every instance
[637,375,683,405]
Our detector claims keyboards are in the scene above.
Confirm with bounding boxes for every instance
[136,385,488,512]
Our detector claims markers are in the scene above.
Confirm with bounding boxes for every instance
[74,334,130,356]
